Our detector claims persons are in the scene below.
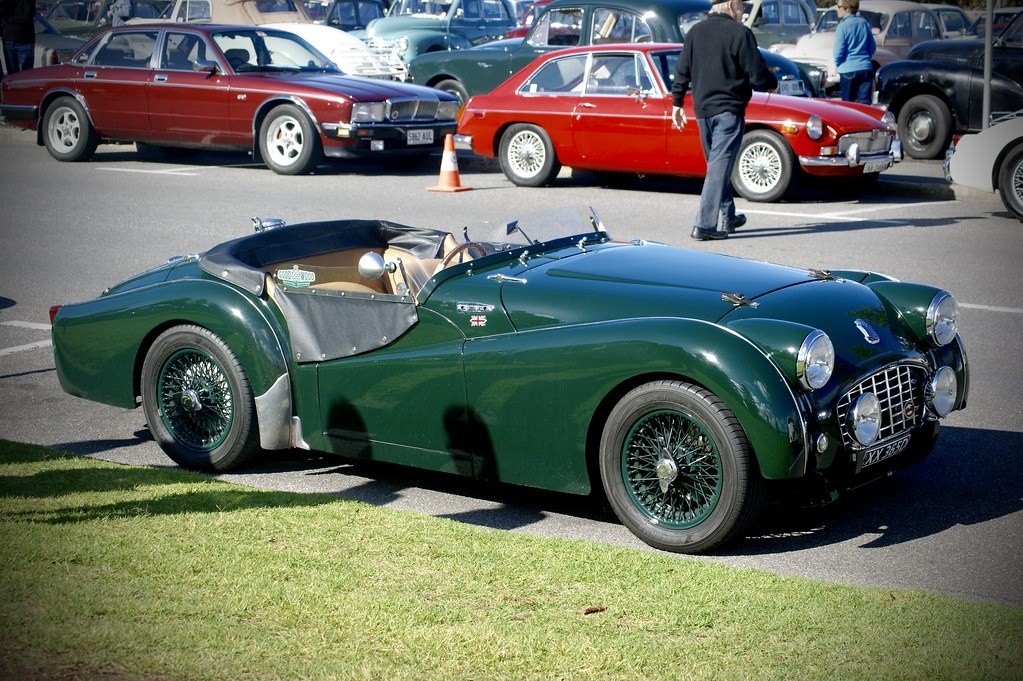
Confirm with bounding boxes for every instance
[107,0,132,28]
[0,0,37,76]
[832,0,877,105]
[671,0,780,242]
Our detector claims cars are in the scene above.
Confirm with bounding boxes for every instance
[0,0,171,76]
[49,201,971,554]
[155,0,313,25]
[767,0,945,82]
[405,0,714,105]
[942,110,1023,218]
[455,42,898,202]
[920,3,986,39]
[0,22,462,175]
[345,0,516,66]
[874,7,1023,159]
[302,0,385,32]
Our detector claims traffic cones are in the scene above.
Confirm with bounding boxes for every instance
[428,133,473,192]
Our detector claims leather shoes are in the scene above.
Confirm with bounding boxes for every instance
[722,213,746,233]
[691,226,729,240]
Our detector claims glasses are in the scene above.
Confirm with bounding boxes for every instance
[837,4,844,8]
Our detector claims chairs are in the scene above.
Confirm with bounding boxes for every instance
[380,230,474,308]
[224,49,254,70]
[166,48,193,71]
[589,74,652,94]
[97,47,140,67]
